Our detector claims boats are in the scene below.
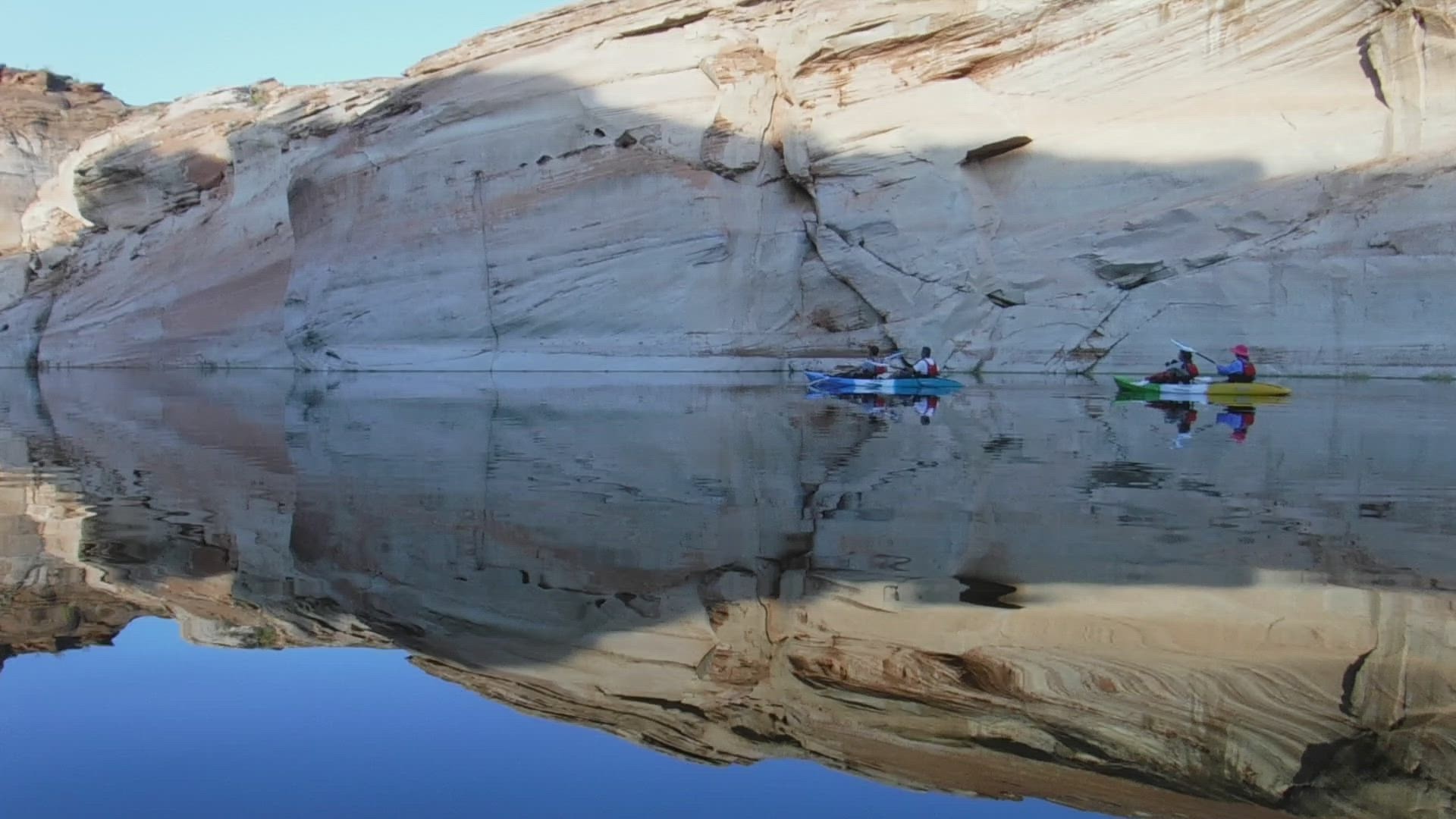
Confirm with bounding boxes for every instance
[1110,390,1293,406]
[1114,374,1293,397]
[802,368,965,389]
[804,384,962,397]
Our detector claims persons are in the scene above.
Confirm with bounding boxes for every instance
[904,346,940,378]
[1165,350,1199,385]
[1215,406,1255,443]
[912,395,939,426]
[1162,407,1197,434]
[1216,344,1256,383]
[845,345,881,375]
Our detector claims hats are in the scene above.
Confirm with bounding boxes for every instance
[1230,345,1249,356]
[1232,432,1245,442]
[867,344,879,355]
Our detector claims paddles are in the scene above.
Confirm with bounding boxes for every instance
[865,360,906,369]
[806,370,851,386]
[1126,379,1148,388]
[1169,337,1219,365]
